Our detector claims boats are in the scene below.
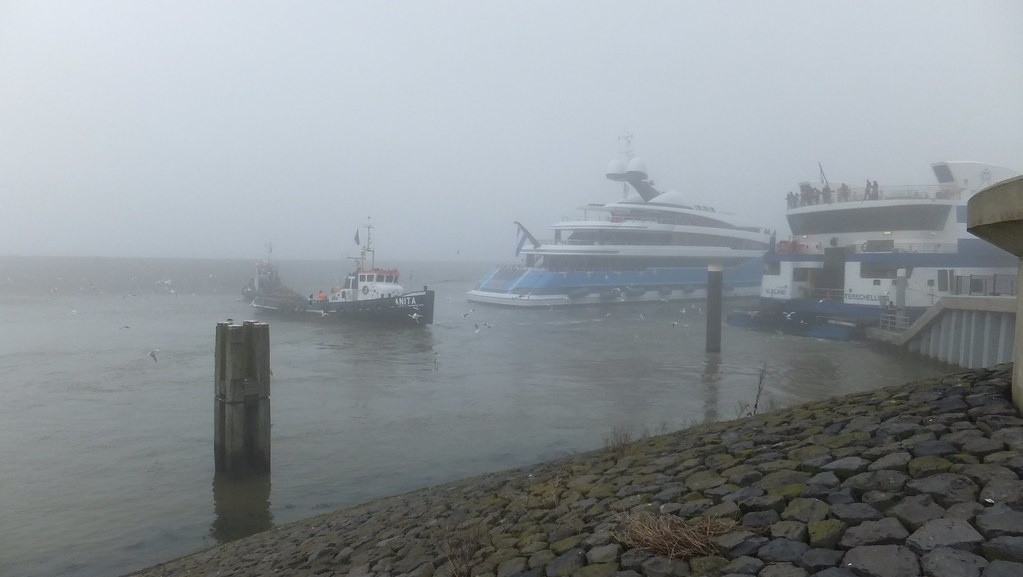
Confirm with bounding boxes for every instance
[238,214,437,329]
[460,156,785,326]
[707,159,1023,347]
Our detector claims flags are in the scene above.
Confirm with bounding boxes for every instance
[354,229,360,245]
[516,225,526,258]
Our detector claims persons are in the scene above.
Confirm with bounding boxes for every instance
[764,228,776,237]
[785,179,879,210]
[318,290,326,300]
[886,301,896,328]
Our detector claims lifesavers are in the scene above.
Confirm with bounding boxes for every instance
[611,287,621,298]
[362,285,369,294]
[944,190,952,199]
[860,242,872,252]
